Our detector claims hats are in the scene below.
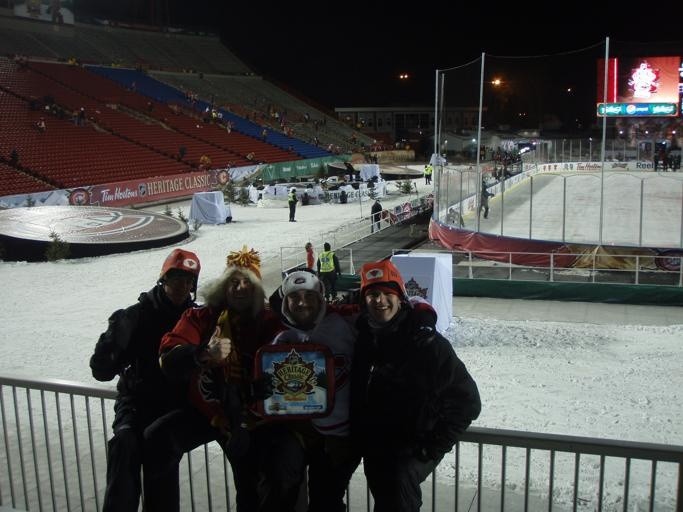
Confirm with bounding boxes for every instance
[355,261,414,323]
[156,248,201,305]
[203,244,266,321]
[278,266,329,341]
[290,186,297,193]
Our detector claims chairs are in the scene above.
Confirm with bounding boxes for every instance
[154,75,376,178]
[155,39,251,76]
[0,29,154,197]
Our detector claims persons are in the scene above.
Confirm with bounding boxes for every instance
[287,187,298,222]
[479,185,495,219]
[352,259,483,512]
[252,268,438,511]
[317,243,342,302]
[141,248,271,512]
[85,249,203,512]
[370,200,382,232]
[303,243,318,273]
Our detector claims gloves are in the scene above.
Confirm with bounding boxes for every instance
[412,429,454,465]
